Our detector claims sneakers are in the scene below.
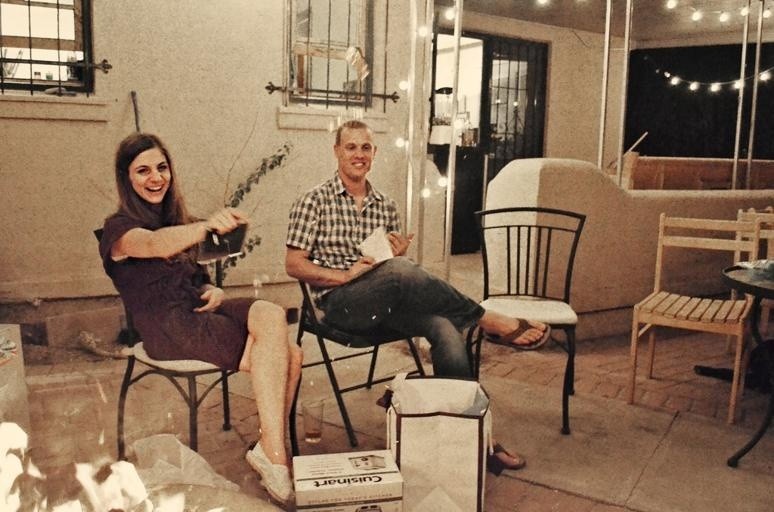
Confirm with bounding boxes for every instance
[243,442,294,505]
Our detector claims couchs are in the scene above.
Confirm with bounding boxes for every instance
[481,157,774,316]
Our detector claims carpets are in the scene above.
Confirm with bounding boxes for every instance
[626,411,774,512]
[196,323,420,414]
[296,363,679,507]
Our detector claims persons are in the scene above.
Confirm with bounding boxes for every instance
[283,118,550,472]
[98,132,305,508]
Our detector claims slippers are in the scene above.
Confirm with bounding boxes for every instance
[483,312,553,352]
[488,443,526,470]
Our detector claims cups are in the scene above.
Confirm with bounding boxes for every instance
[301,399,324,443]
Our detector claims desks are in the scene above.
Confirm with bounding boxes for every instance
[721,259,774,468]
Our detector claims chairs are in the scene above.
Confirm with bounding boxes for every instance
[93,228,302,462]
[289,280,424,450]
[724,209,774,350]
[749,205,774,261]
[626,212,760,426]
[464,205,587,434]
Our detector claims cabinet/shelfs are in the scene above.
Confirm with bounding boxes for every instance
[427,142,484,254]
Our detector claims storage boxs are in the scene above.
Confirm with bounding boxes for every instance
[292,444,405,512]
